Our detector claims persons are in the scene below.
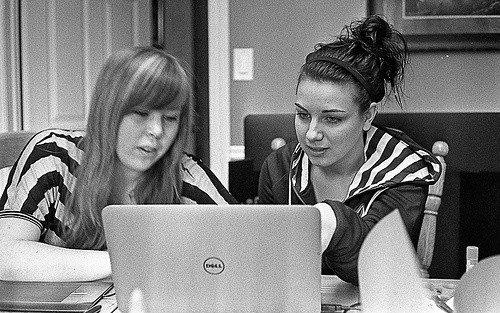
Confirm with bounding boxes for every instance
[0,45,238,283]
[259,14,442,286]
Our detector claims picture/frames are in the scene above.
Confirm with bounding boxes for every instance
[367,0,500,53]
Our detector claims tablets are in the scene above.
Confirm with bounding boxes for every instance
[0,280,113,313]
[101,203,348,313]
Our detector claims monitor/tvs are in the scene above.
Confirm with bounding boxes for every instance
[386,0,500,36]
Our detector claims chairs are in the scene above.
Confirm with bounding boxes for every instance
[271,138,449,279]
